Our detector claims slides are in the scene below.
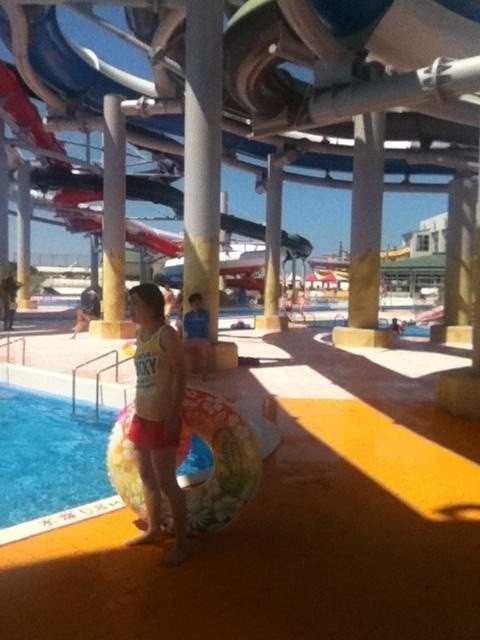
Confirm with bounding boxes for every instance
[2,127,313,305]
[1,1,458,193]
[0,59,288,300]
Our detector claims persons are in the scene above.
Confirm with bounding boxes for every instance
[161,282,174,326]
[181,293,214,384]
[71,289,102,332]
[0,276,24,331]
[175,287,183,333]
[389,317,405,336]
[124,282,192,570]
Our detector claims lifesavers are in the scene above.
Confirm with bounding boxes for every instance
[106,386,259,534]
